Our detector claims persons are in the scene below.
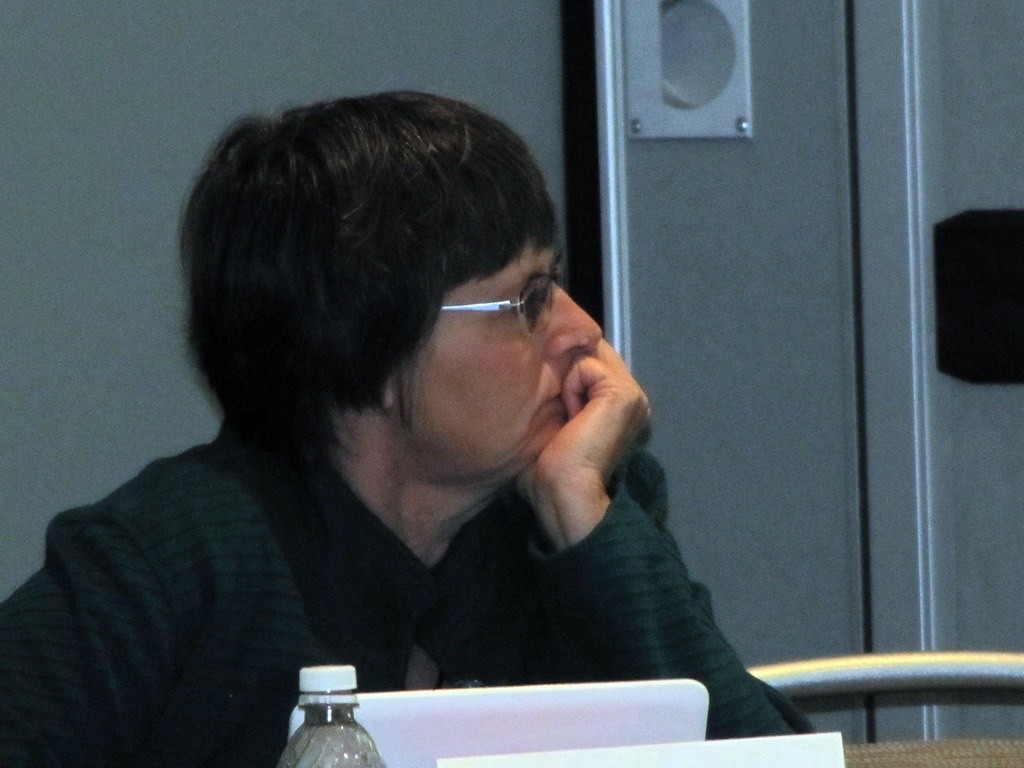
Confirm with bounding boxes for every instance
[0,89,815,768]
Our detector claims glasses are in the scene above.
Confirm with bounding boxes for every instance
[440,249,572,336]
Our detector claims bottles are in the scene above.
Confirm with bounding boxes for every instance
[279,664,384,768]
